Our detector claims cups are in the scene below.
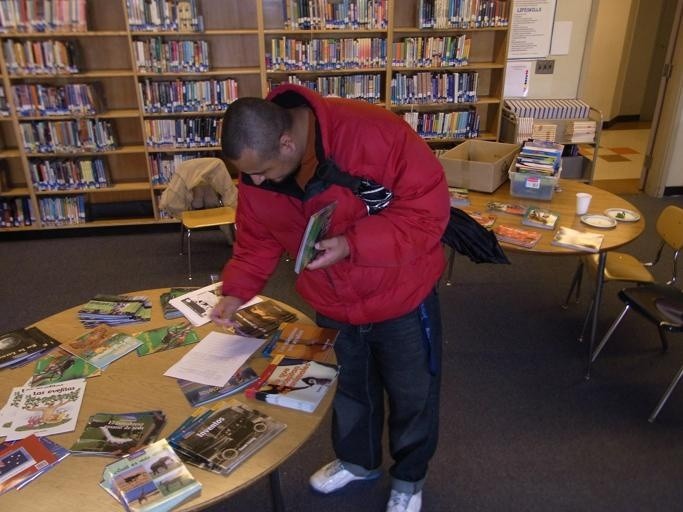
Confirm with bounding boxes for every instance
[574,192,591,213]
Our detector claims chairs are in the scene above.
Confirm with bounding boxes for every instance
[177,181,237,280]
[559,202,682,352]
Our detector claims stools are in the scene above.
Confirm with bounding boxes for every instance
[585,282,682,424]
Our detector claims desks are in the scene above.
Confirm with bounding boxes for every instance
[0,284,339,511]
[444,177,646,383]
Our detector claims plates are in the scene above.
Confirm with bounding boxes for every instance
[604,207,640,223]
[580,215,616,229]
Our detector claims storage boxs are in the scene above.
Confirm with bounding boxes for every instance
[436,136,520,194]
[506,152,563,202]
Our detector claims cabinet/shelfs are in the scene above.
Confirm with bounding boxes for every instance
[0,72,39,231]
[499,105,604,184]
[259,0,389,117]
[390,1,514,155]
[0,0,158,231]
[123,0,265,226]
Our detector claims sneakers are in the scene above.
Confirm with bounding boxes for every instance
[308,456,379,493]
[386,488,423,511]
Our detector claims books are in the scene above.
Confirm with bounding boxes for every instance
[1,281,342,512]
[144,117,223,218]
[2,157,111,230]
[295,200,336,275]
[448,187,603,254]
[505,98,596,143]
[126,1,210,74]
[392,1,510,68]
[139,77,239,113]
[267,1,388,102]
[391,72,480,141]
[1,82,121,153]
[1,2,89,76]
[515,138,565,176]
